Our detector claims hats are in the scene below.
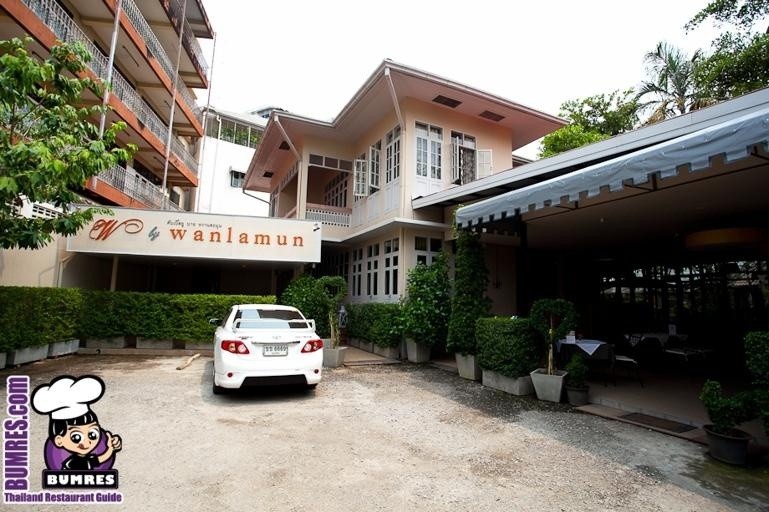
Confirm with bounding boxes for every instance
[29,373,105,422]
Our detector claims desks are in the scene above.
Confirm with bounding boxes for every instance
[556,338,607,383]
[624,331,686,352]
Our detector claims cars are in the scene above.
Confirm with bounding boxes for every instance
[204,301,322,393]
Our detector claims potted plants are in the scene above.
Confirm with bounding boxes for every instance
[445,230,591,406]
[314,276,349,368]
[347,302,443,364]
[699,378,760,466]
[0,298,217,368]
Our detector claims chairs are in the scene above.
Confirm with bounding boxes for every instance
[600,333,646,388]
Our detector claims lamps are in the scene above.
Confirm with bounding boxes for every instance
[337,305,348,329]
